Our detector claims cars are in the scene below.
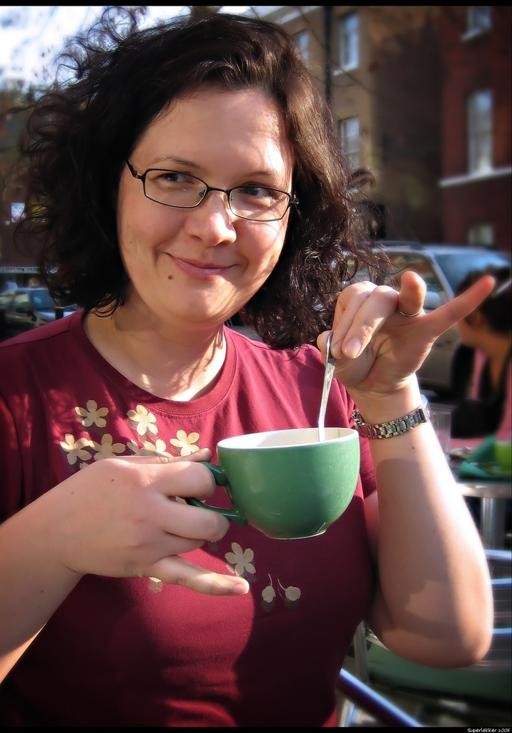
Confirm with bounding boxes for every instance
[325,241,512,403]
[0,284,88,339]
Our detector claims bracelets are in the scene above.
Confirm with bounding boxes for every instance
[351,393,431,441]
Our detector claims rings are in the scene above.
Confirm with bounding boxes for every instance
[397,307,422,320]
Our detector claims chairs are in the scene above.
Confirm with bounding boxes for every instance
[335,550,512,727]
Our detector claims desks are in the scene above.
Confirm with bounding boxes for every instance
[446,438,512,550]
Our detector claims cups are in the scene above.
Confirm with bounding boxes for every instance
[183,427,360,543]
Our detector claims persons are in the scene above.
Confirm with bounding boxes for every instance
[0,12,499,731]
[434,263,512,450]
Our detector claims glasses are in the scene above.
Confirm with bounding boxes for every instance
[124,156,299,222]
